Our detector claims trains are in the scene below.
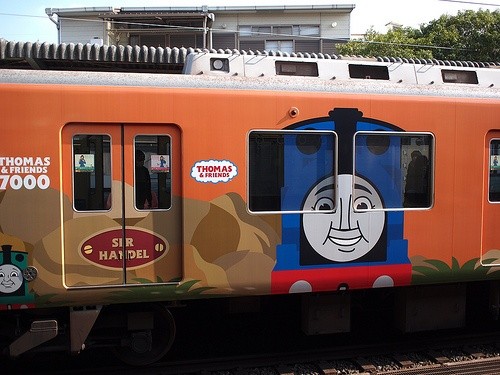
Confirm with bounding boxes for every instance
[1,40,500,312]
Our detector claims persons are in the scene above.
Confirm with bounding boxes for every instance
[403,156,430,208]
[492,156,498,166]
[404,151,422,183]
[159,155,167,167]
[135,149,153,209]
[78,155,86,167]
